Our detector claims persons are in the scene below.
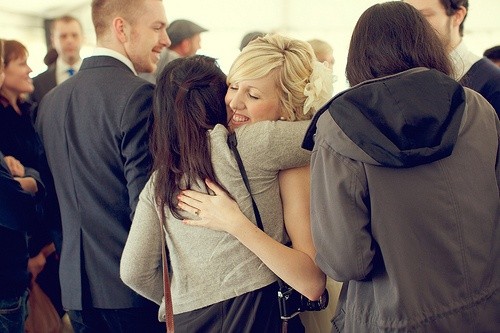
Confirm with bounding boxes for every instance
[120,54,313,333]
[301,1,500,333]
[177,33,343,333]
[138,1,500,119]
[33,0,171,333]
[0,16,86,333]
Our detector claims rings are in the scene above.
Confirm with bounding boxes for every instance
[195,210,200,218]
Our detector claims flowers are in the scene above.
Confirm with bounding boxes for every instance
[302,60,337,118]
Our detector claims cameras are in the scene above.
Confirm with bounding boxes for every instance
[277,284,329,320]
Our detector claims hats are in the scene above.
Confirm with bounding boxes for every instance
[166,20,207,47]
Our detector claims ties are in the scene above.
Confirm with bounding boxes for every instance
[67,69,74,76]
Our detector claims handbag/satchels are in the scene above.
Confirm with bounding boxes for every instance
[277,289,305,333]
[23,280,62,333]
